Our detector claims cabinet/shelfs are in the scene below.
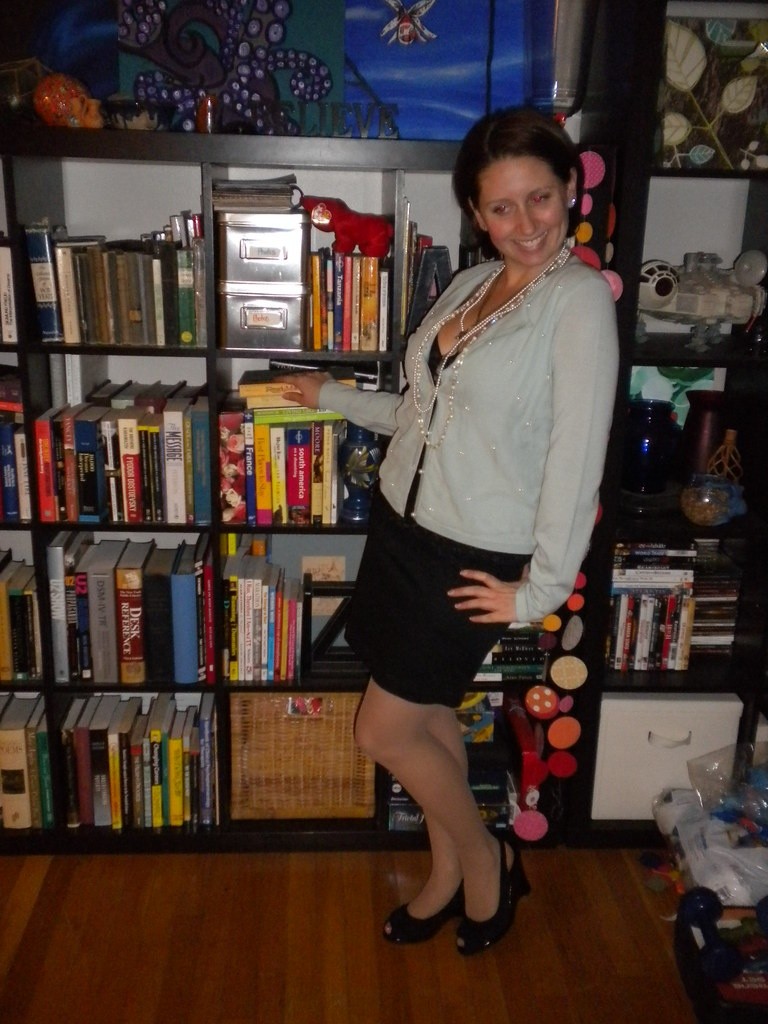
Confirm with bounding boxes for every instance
[0,0,632,859]
[562,0,768,853]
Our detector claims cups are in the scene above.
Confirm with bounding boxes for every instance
[680,472,739,528]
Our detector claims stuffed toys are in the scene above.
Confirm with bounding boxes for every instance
[300,194,393,256]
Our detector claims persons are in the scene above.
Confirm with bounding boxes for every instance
[277,104,618,955]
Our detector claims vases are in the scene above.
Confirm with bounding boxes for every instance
[630,388,747,528]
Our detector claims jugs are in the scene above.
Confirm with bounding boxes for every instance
[618,401,685,520]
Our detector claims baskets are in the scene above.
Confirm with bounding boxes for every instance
[230,692,376,820]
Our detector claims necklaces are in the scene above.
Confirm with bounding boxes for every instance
[413,243,571,450]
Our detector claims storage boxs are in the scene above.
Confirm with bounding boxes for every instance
[654,17,768,171]
[216,213,314,350]
[593,692,746,820]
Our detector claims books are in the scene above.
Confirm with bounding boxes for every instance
[0,352,211,527]
[473,620,550,681]
[222,531,304,681]
[304,246,392,352]
[0,530,214,684]
[217,359,378,526]
[0,214,208,347]
[606,533,741,673]
[0,691,218,829]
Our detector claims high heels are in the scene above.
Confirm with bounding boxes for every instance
[384,879,463,943]
[456,838,530,954]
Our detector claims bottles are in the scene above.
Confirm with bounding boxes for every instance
[680,388,731,473]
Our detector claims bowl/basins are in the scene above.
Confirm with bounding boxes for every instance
[103,99,181,132]
